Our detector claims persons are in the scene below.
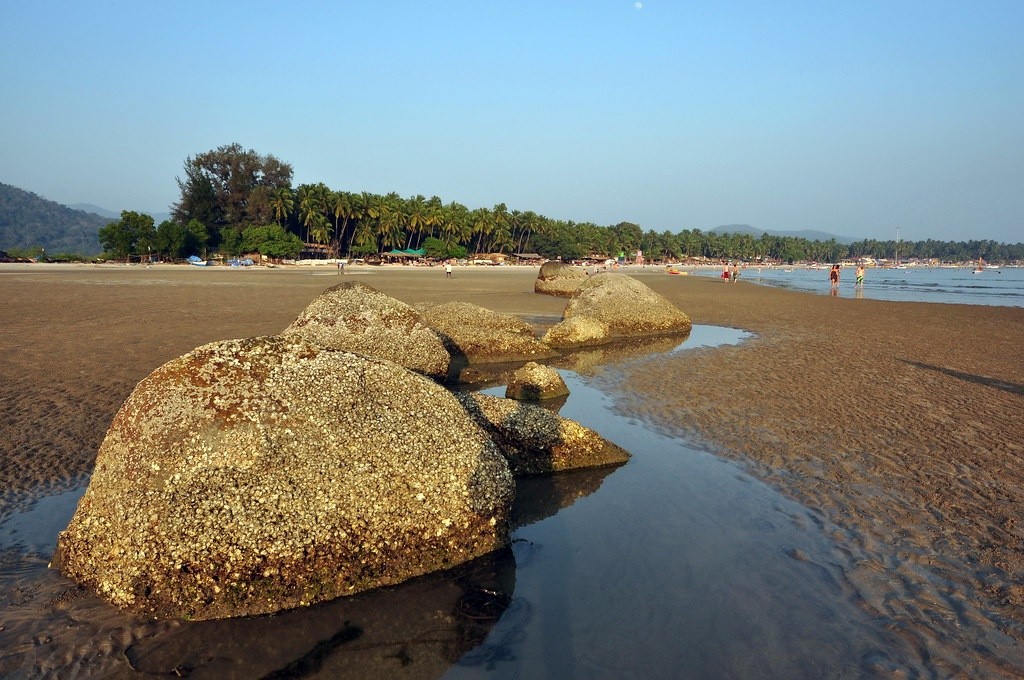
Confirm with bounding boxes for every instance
[338,259,982,289]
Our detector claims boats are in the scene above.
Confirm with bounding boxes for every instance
[192,261,208,266]
